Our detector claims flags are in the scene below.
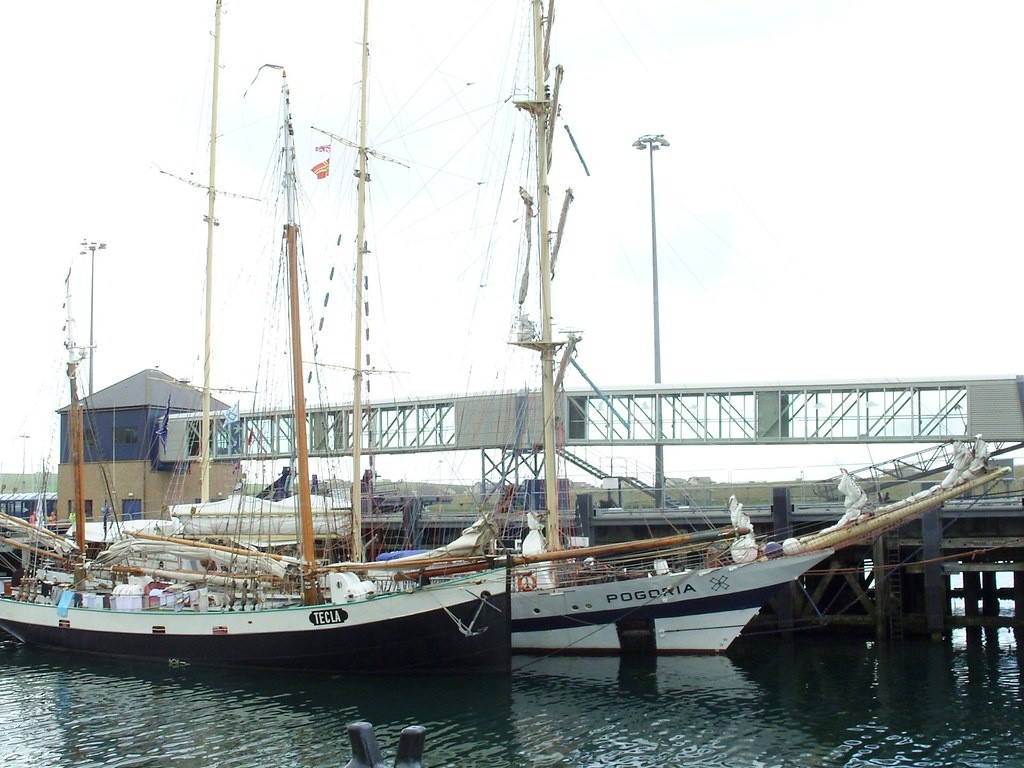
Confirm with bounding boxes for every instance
[314,144,331,153]
[232,458,240,474]
[248,427,254,446]
[310,158,330,179]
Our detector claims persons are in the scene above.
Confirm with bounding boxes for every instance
[24,509,76,535]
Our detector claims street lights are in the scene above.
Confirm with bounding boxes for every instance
[632,133,670,384]
[20,435,31,494]
[78,240,107,396]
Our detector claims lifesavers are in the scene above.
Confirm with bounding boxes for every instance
[518,575,537,591]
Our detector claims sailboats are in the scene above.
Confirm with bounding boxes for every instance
[0,221,517,680]
[1,1,1023,650]
[509,652,775,698]
